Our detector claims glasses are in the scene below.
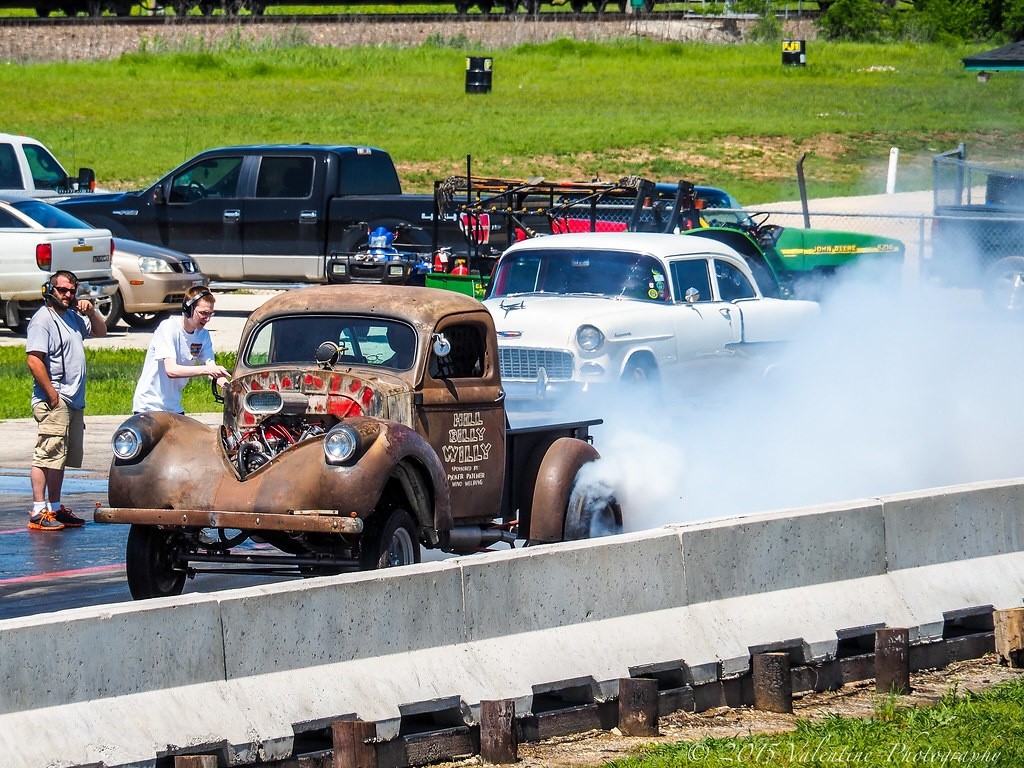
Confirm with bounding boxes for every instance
[53,285,77,295]
[195,309,216,317]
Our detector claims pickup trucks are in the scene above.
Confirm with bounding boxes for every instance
[49,142,552,286]
[0,225,119,327]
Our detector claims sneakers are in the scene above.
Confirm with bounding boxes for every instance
[48,504,86,527]
[28,508,65,531]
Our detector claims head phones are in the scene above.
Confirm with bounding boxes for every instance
[182,291,212,318]
[41,271,78,304]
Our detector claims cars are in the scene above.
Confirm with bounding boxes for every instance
[0,195,212,335]
[477,230,823,429]
[93,285,626,607]
[553,182,760,233]
[0,134,115,207]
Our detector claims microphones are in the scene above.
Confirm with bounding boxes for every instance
[43,292,61,305]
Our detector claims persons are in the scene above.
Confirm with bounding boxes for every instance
[132,286,232,414]
[25,269,107,531]
